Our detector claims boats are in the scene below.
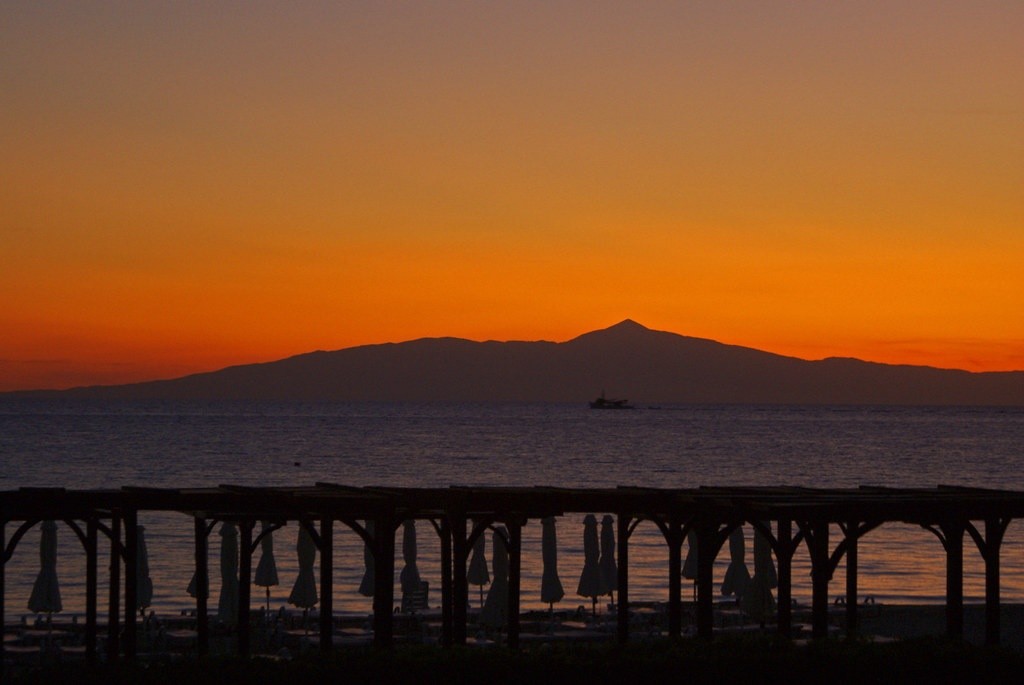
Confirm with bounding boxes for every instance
[588,389,629,409]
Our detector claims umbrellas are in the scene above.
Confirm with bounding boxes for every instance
[681,531,698,602]
[218,523,240,626]
[400,520,421,614]
[288,519,318,637]
[541,517,564,634]
[469,519,507,646]
[136,524,152,619]
[28,519,62,649]
[722,519,778,630]
[576,515,618,621]
[359,519,375,596]
[255,519,278,628]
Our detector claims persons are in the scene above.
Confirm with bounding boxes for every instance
[279,606,287,617]
[71,617,81,634]
[34,615,43,630]
